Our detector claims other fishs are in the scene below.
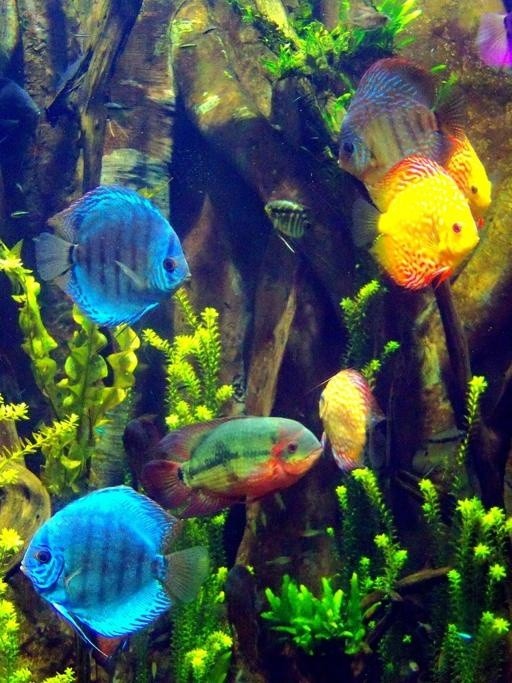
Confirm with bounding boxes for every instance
[0,24,332,220]
